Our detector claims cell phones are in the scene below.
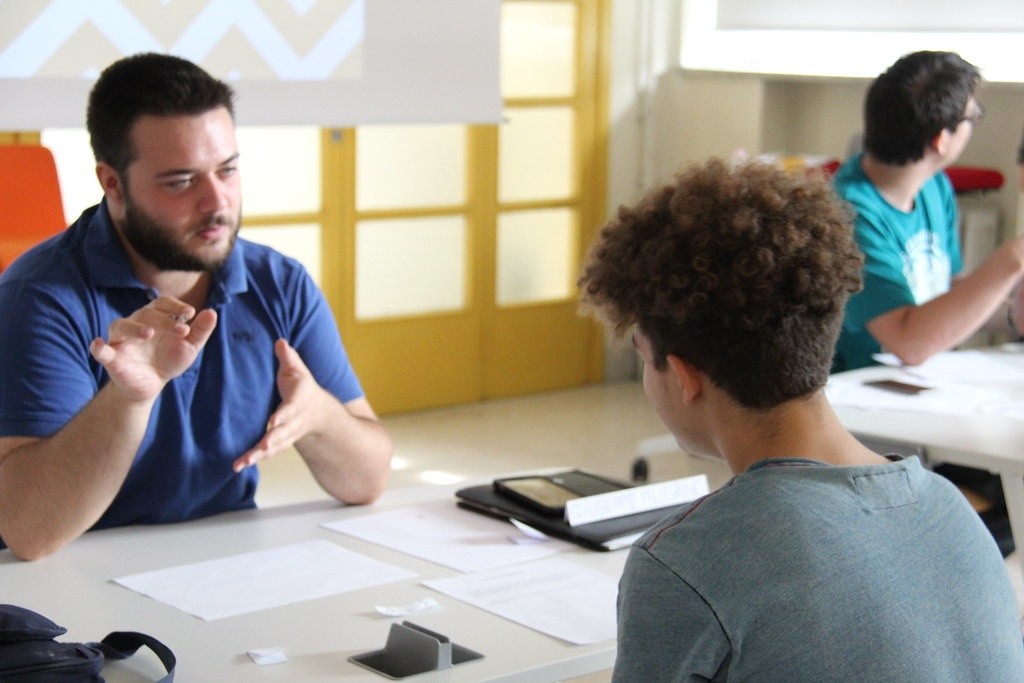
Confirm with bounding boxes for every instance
[493,476,581,518]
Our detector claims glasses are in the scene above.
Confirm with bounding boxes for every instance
[957,102,986,127]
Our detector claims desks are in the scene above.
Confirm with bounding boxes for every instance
[824,340,1024,571]
[0,433,734,683]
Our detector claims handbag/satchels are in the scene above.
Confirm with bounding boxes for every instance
[0,605,176,683]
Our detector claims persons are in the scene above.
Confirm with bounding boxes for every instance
[0,51,393,562]
[573,150,1024,683]
[829,50,1024,517]
